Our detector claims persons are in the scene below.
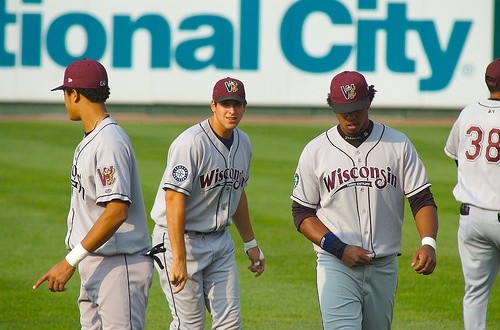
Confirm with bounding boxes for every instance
[33,58,154,330]
[444,57,500,330]
[290,70,438,330]
[150,77,266,330]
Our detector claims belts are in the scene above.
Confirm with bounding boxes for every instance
[460,206,500,223]
[184,230,217,234]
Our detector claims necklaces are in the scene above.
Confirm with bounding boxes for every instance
[93,112,110,128]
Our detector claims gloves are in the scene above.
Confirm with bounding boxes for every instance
[143,243,166,269]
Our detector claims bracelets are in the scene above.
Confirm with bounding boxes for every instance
[65,243,91,267]
[319,231,347,260]
[420,237,436,252]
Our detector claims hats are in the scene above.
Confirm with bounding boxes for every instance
[330,71,370,113]
[213,77,246,103]
[485,58,500,87]
[51,58,108,91]
